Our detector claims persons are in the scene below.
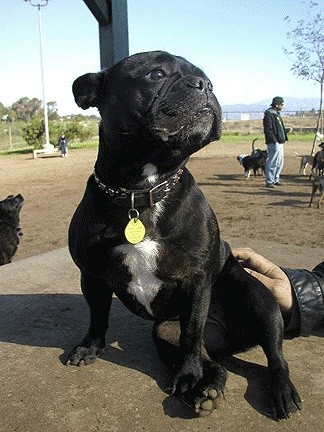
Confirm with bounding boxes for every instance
[58,132,67,157]
[232,247,323,339]
[263,96,292,189]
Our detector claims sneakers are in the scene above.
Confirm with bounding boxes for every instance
[275,182,286,186]
[265,183,276,188]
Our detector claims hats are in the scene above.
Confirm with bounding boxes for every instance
[269,97,284,106]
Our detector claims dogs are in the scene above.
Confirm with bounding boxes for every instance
[0,194,24,267]
[295,150,322,177]
[250,137,275,160]
[309,172,324,209]
[236,153,266,180]
[312,140,324,176]
[64,50,301,422]
